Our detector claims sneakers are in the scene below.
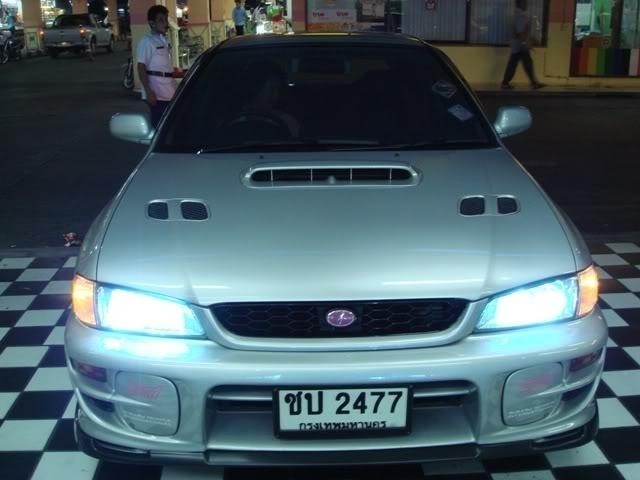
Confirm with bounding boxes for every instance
[501,82,513,89]
[533,83,544,89]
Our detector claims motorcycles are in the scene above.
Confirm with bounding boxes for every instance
[0,25,26,64]
[122,33,134,89]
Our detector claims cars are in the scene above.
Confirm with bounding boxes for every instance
[65,29,609,468]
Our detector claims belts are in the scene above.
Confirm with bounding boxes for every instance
[147,71,173,77]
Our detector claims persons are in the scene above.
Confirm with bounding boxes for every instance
[232,1,250,35]
[219,60,304,138]
[500,0,548,88]
[136,4,188,126]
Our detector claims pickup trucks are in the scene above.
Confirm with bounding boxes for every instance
[40,12,116,60]
